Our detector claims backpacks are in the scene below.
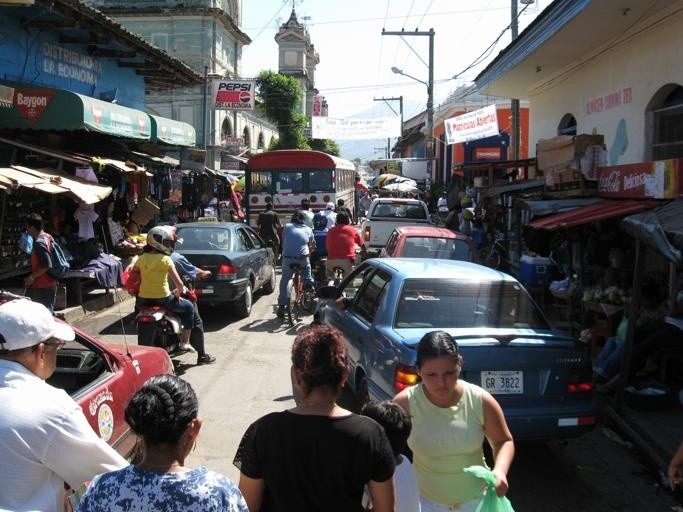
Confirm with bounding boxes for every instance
[34,233,70,281]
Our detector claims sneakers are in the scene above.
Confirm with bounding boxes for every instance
[195,353,217,367]
[178,341,196,354]
[277,307,285,320]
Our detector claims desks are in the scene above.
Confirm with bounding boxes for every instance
[528,285,546,310]
[62,256,124,303]
[114,241,147,260]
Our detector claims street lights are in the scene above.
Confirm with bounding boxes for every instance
[390,66,434,197]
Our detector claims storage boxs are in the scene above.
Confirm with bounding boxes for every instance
[520,254,554,282]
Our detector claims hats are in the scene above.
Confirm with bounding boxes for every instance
[0,296,75,352]
[325,202,335,210]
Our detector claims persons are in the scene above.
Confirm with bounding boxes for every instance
[0,297,131,512]
[666,439,683,491]
[128,175,460,365]
[23,213,59,315]
[232,323,396,512]
[390,330,516,512]
[75,372,250,512]
[359,400,421,512]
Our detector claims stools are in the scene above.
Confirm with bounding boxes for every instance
[659,346,681,380]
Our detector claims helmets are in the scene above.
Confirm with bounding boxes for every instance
[146,225,178,257]
[311,213,328,230]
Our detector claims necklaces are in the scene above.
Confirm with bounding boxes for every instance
[297,398,338,408]
[139,460,181,469]
